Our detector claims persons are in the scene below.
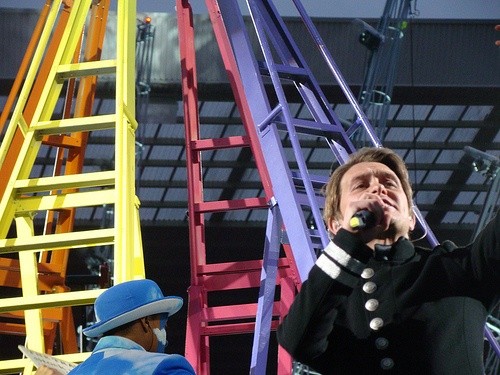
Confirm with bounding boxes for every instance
[67,279,196,375]
[277,146,500,375]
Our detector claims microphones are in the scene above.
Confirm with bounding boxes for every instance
[349,203,385,230]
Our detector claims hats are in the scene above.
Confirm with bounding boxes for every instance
[81,278,184,339]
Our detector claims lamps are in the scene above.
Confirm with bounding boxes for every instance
[472,160,486,172]
[359,30,381,50]
